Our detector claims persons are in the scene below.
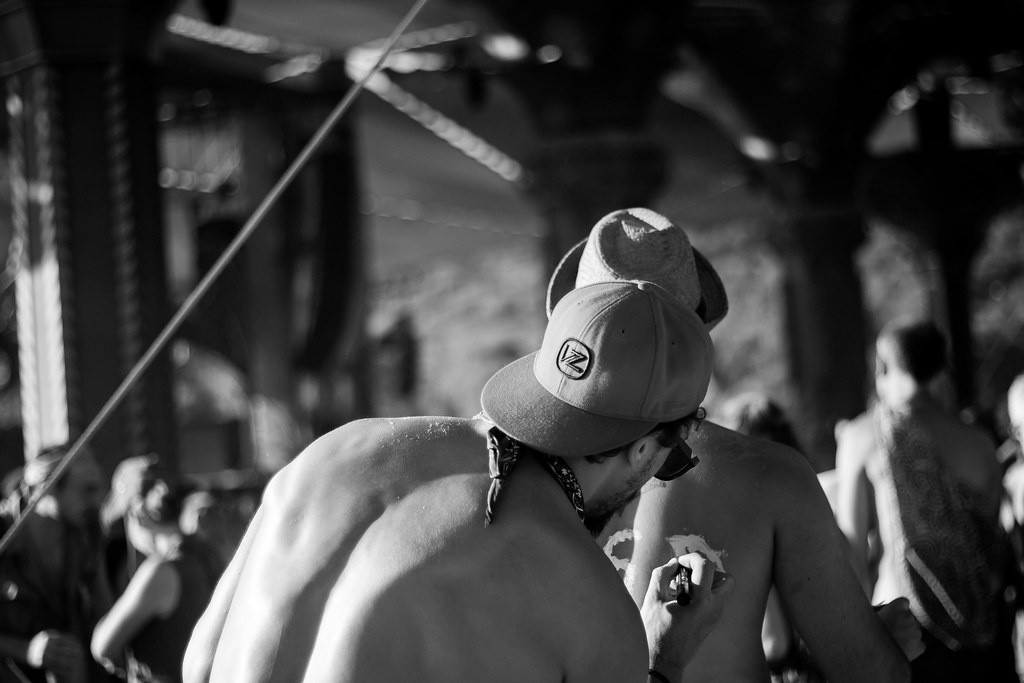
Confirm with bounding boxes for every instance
[722,313,1024,683]
[546,207,926,683]
[0,445,223,683]
[180,279,727,683]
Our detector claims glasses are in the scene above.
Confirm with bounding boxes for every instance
[653,422,699,482]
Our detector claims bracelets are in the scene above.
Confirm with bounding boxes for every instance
[648,668,670,683]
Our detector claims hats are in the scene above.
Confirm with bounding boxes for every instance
[480,279,717,459]
[546,207,728,331]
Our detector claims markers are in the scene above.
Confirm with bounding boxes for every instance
[674,565,692,606]
